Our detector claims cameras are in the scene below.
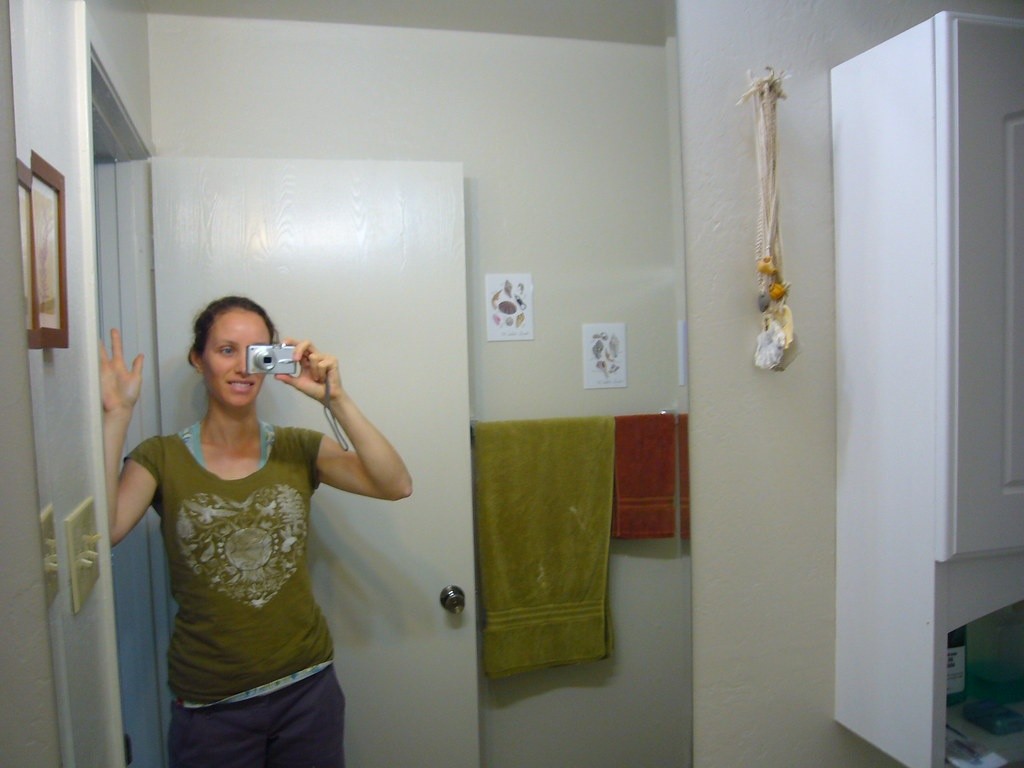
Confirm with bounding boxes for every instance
[247,344,298,375]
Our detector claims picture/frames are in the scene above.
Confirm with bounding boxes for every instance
[16,159,40,349]
[30,149,69,348]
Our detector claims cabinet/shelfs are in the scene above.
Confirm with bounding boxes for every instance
[830,12,1024,768]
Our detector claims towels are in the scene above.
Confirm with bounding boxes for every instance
[473,416,617,677]
[612,409,693,540]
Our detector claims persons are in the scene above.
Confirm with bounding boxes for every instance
[97,296,414,768]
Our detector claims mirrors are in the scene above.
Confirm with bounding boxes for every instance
[9,1,1024,768]
